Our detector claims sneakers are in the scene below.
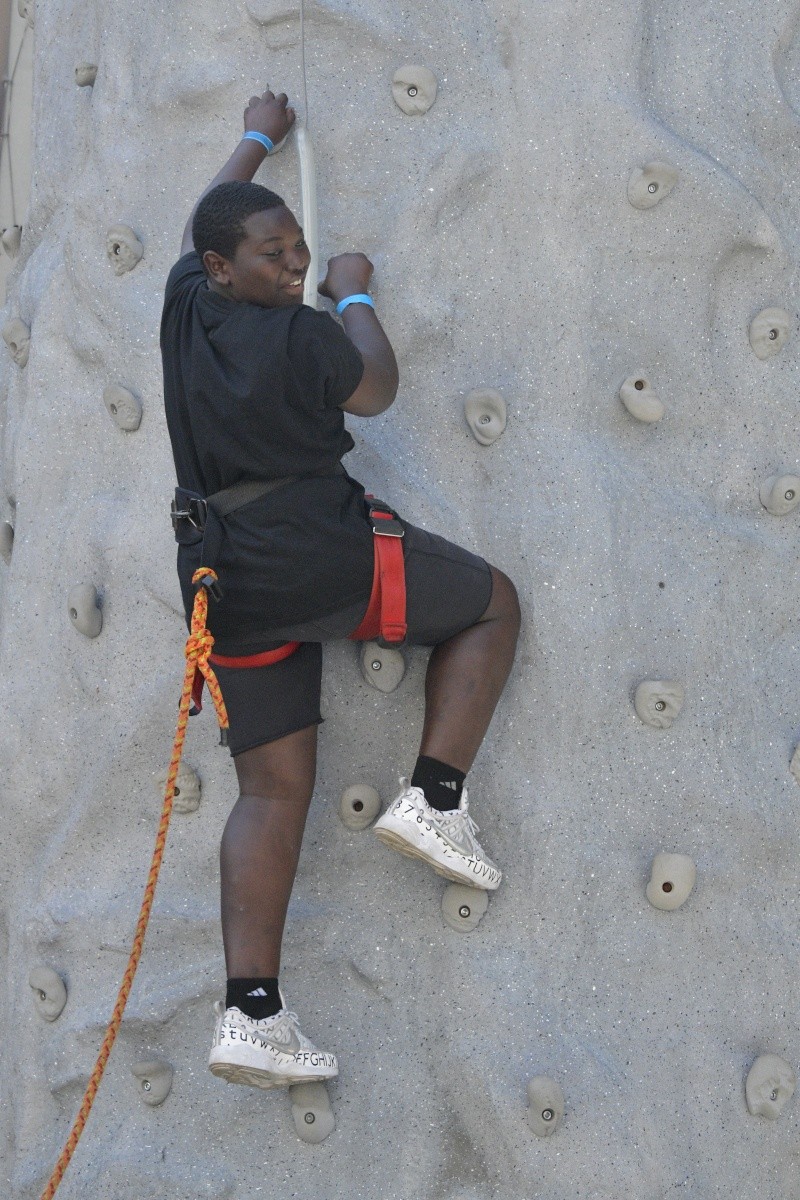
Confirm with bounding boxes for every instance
[207,984,338,1090]
[371,777,504,890]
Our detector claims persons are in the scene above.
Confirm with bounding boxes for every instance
[159,91,521,1087]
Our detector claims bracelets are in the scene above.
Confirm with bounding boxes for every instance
[241,130,275,152]
[334,294,376,315]
